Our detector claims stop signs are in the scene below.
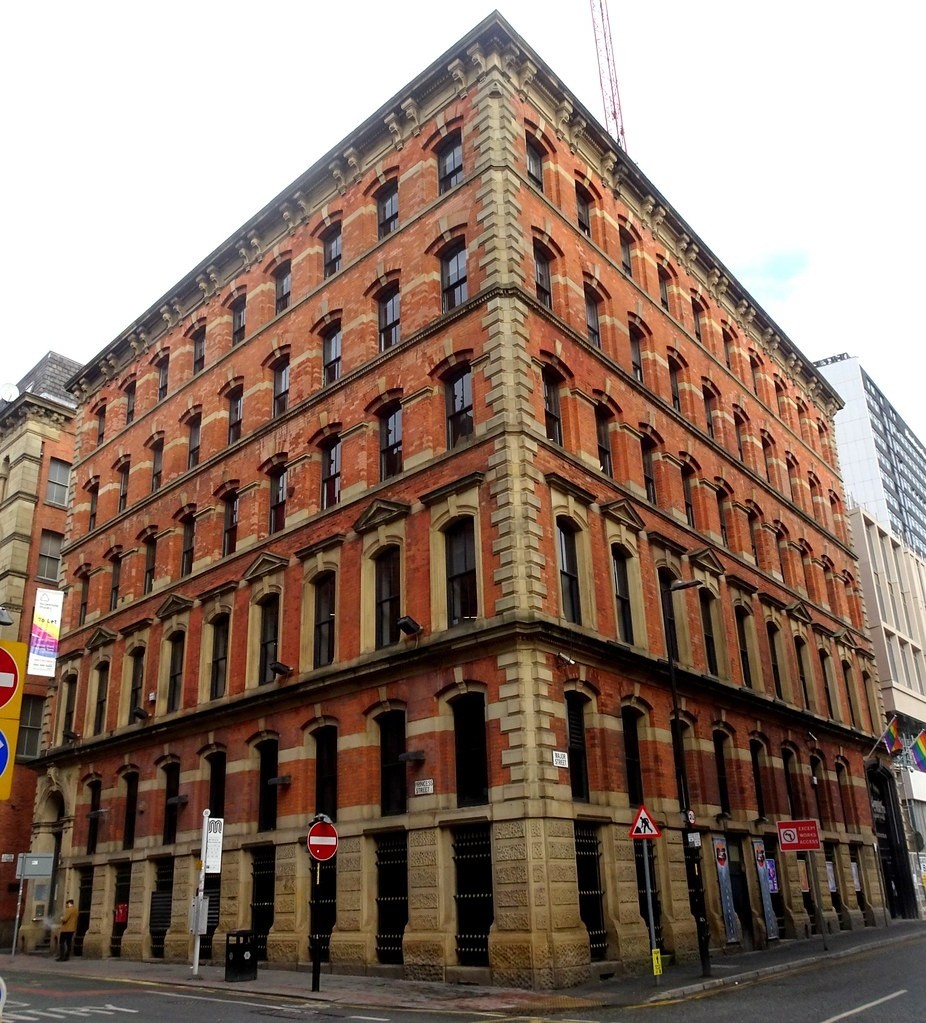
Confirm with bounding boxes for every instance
[0,640,28,720]
[307,821,339,862]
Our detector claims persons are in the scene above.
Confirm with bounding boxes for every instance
[55,899,79,962]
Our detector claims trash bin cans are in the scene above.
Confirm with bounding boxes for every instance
[225,929,257,982]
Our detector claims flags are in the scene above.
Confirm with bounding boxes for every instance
[880,714,903,756]
[910,730,926,773]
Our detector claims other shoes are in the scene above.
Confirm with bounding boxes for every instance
[56,956,69,961]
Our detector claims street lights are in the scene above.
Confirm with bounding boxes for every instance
[666,575,714,978]
